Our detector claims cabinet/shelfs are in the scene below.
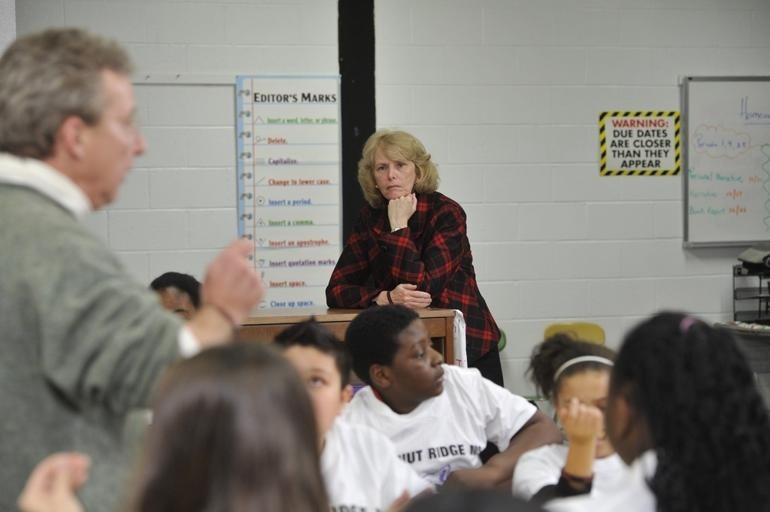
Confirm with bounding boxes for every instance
[732,263,768,325]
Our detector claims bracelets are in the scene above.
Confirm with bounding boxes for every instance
[561,464,595,485]
[390,223,408,234]
[198,299,246,333]
[386,291,394,305]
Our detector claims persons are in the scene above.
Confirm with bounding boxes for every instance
[148,271,203,324]
[511,330,632,502]
[324,127,506,389]
[265,313,432,511]
[542,311,769,511]
[0,24,269,511]
[12,338,331,512]
[400,482,551,512]
[341,301,567,492]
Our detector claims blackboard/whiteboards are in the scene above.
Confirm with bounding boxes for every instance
[679,76,770,249]
